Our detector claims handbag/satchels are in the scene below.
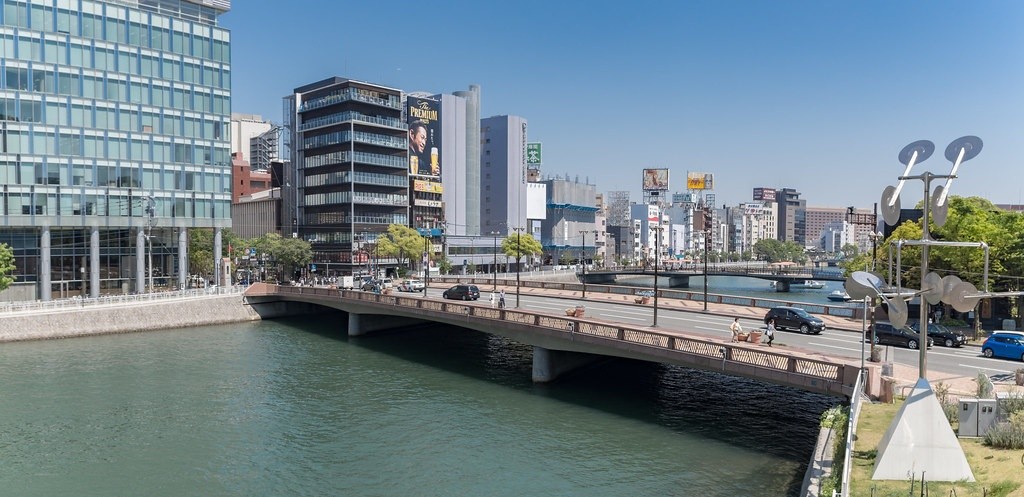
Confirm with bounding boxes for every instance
[731,332,734,336]
[765,330,767,335]
[730,323,735,330]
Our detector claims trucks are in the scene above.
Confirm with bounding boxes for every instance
[337,276,353,290]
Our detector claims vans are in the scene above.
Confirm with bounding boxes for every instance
[443,285,480,301]
[362,278,392,291]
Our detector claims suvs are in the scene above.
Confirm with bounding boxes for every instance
[763,306,825,334]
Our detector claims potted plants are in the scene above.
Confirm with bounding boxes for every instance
[750,329,762,344]
[738,332,750,341]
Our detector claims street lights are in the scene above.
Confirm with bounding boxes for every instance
[355,234,364,290]
[513,228,525,307]
[651,225,664,327]
[700,230,711,310]
[579,231,588,298]
[491,231,500,291]
[421,229,433,297]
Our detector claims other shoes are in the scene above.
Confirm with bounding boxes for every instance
[767,342,770,344]
[737,341,740,343]
[732,341,735,343]
[769,345,772,347]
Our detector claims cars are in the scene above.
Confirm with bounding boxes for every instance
[981,330,1024,361]
[866,322,933,349]
[910,323,967,348]
[398,280,424,293]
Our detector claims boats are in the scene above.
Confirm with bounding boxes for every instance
[827,291,852,301]
[804,280,824,289]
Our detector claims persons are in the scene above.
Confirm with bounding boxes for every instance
[409,120,440,175]
[765,319,777,347]
[499,288,506,308]
[488,290,495,308]
[309,274,337,285]
[729,317,743,343]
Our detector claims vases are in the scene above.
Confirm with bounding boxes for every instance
[634,298,642,304]
[575,305,585,317]
[643,297,650,304]
[565,308,576,316]
